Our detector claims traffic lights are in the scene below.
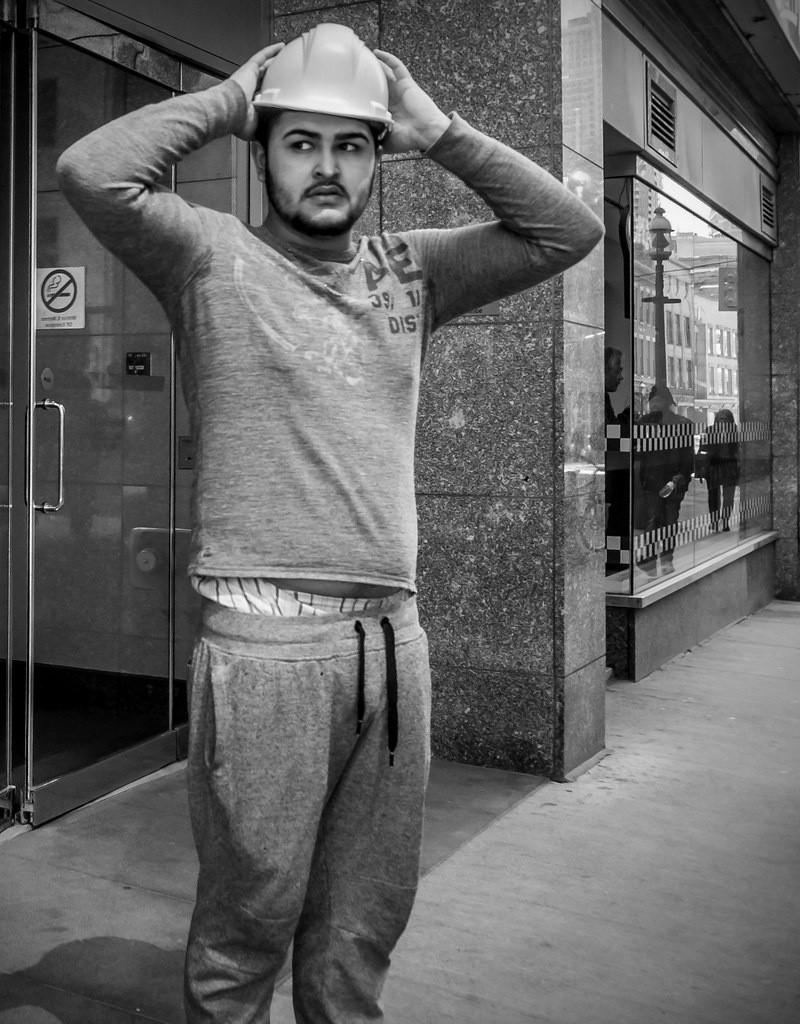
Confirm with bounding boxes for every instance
[718,267,737,311]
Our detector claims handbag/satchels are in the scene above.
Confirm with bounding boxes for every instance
[695,448,707,479]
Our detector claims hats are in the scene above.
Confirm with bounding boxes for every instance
[648,384,676,404]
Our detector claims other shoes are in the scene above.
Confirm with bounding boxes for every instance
[709,522,719,532]
[661,562,675,574]
[723,518,730,532]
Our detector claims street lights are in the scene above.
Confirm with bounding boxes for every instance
[641,206,682,423]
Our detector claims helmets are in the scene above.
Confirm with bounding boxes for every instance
[251,23,395,126]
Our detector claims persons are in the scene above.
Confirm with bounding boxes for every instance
[696,411,739,532]
[56,23,603,1023]
[604,348,695,579]
